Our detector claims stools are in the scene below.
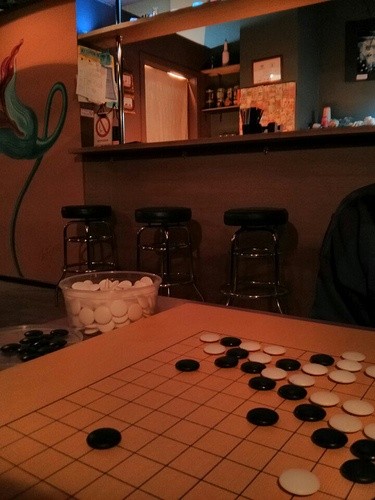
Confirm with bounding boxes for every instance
[220,208,289,315]
[134,206,206,302]
[54,203,122,308]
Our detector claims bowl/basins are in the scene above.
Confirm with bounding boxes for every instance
[58,272,163,340]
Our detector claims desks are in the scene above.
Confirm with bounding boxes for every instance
[0,296,375,377]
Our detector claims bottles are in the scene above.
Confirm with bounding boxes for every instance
[222,41,229,67]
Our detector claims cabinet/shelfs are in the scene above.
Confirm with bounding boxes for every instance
[201,63,239,112]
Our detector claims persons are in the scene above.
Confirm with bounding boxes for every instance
[310,183,375,329]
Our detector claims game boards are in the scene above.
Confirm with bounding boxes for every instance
[0,301,375,500]
